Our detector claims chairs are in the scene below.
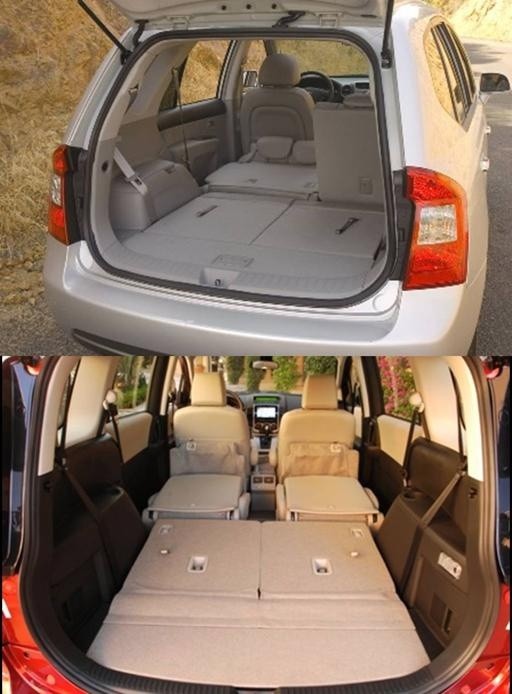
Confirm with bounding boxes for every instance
[241,53,315,155]
[173,371,259,475]
[268,374,360,483]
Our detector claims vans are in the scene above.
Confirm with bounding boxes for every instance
[43,2,511,355]
